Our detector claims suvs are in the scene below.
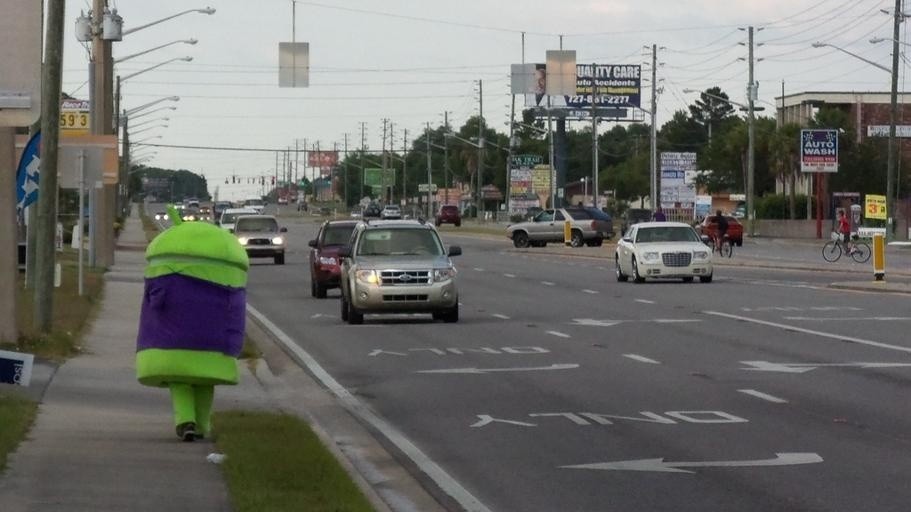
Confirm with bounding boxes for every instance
[308,219,368,300]
[507,206,618,250]
[434,204,462,227]
[350,202,400,220]
[339,218,464,325]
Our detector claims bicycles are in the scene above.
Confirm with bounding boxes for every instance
[822,230,873,263]
[703,229,733,257]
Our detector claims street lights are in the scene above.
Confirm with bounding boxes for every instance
[607,93,657,219]
[81,6,217,268]
[870,37,911,53]
[811,41,899,228]
[543,105,599,207]
[501,120,554,208]
[274,133,513,219]
[682,87,756,220]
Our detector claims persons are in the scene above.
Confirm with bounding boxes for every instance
[532,64,547,95]
[849,199,866,232]
[650,206,667,220]
[837,210,855,256]
[707,209,730,251]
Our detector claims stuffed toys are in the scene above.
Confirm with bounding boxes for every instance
[133,202,250,449]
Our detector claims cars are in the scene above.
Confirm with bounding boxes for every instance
[692,216,743,247]
[156,195,287,265]
[615,222,715,285]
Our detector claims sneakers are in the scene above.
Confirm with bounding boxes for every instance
[182,424,195,442]
